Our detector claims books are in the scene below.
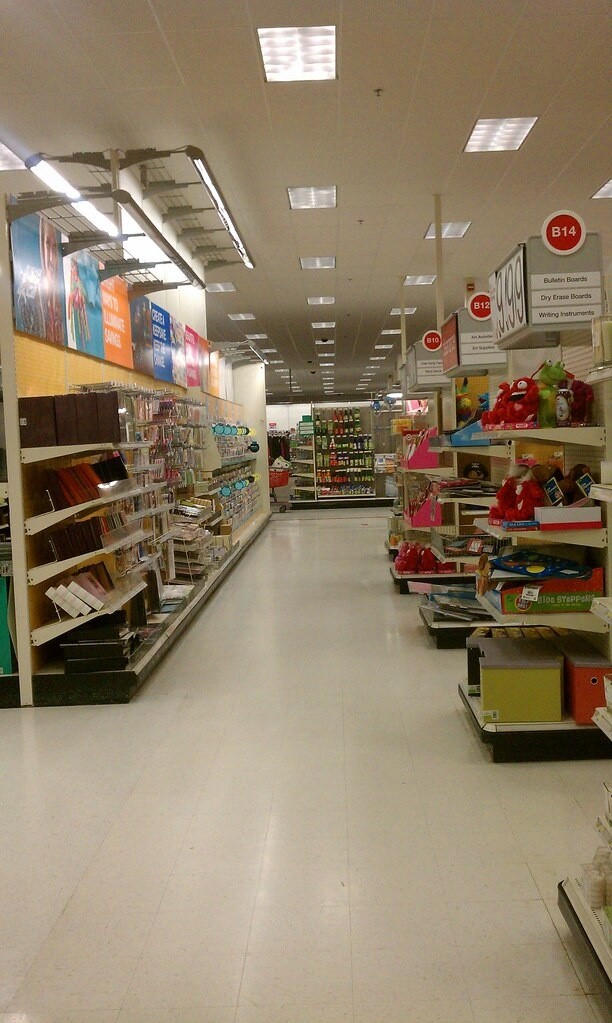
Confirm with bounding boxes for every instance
[18,391,121,448]
[44,560,115,619]
[47,510,131,560]
[44,456,129,511]
[59,611,134,674]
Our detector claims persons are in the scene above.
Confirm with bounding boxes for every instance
[38,217,64,343]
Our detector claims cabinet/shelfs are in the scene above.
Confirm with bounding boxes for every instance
[0,334,272,699]
[291,404,402,501]
[458,366,612,764]
[384,435,458,563]
[422,441,515,649]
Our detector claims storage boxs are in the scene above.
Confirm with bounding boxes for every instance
[503,521,538,532]
[467,628,612,723]
[475,566,605,616]
[210,533,232,549]
[536,507,602,532]
[46,571,110,618]
[18,392,121,448]
[59,609,140,674]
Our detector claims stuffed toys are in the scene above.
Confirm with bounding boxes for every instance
[481,359,594,426]
[488,437,591,522]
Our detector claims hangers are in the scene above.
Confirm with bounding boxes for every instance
[267,428,290,436]
